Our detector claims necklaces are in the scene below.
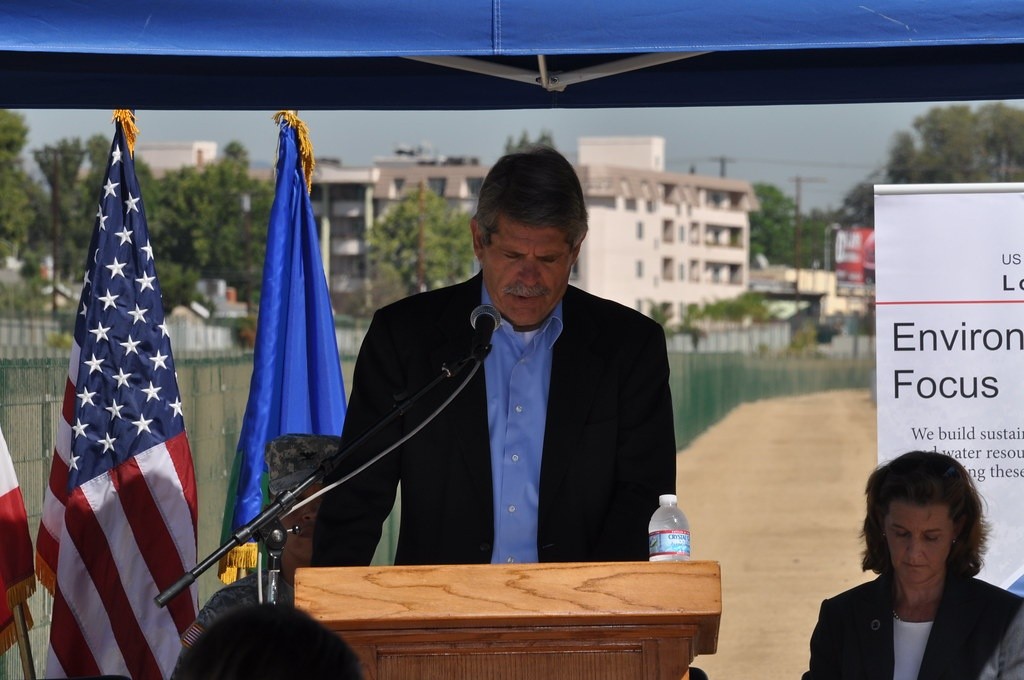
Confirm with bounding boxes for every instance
[893,609,902,621]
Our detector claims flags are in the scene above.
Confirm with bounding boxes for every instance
[218,111,347,585]
[0,427,37,657]
[35,109,200,680]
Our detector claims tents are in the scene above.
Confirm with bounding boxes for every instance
[0,1,1024,115]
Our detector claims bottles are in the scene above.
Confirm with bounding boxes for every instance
[648,494,690,562]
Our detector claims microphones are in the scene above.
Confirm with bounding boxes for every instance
[470,305,502,352]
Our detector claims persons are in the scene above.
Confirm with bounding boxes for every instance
[802,451,1024,680]
[311,147,677,566]
[170,433,364,680]
